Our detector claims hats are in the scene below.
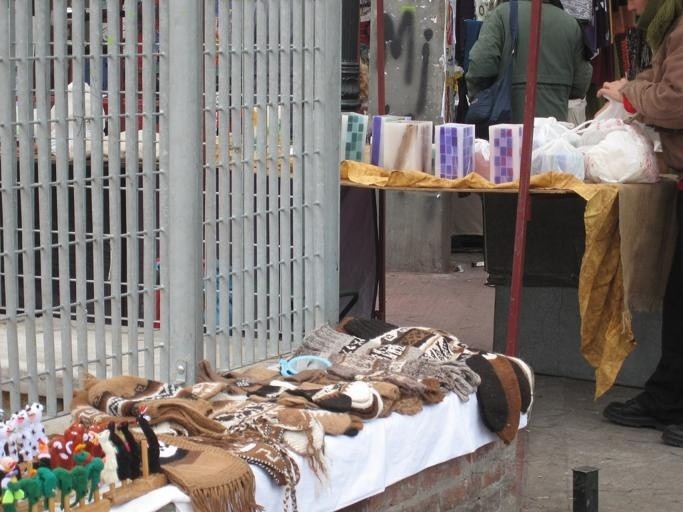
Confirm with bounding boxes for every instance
[81,374,227,433]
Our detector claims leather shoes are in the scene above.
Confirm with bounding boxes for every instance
[662,422,683,447]
[602,396,667,431]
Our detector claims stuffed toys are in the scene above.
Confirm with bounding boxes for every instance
[0,402,160,512]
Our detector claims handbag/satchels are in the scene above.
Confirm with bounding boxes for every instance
[464,62,513,139]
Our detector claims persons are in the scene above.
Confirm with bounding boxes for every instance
[463,1,593,127]
[591,1,682,443]
